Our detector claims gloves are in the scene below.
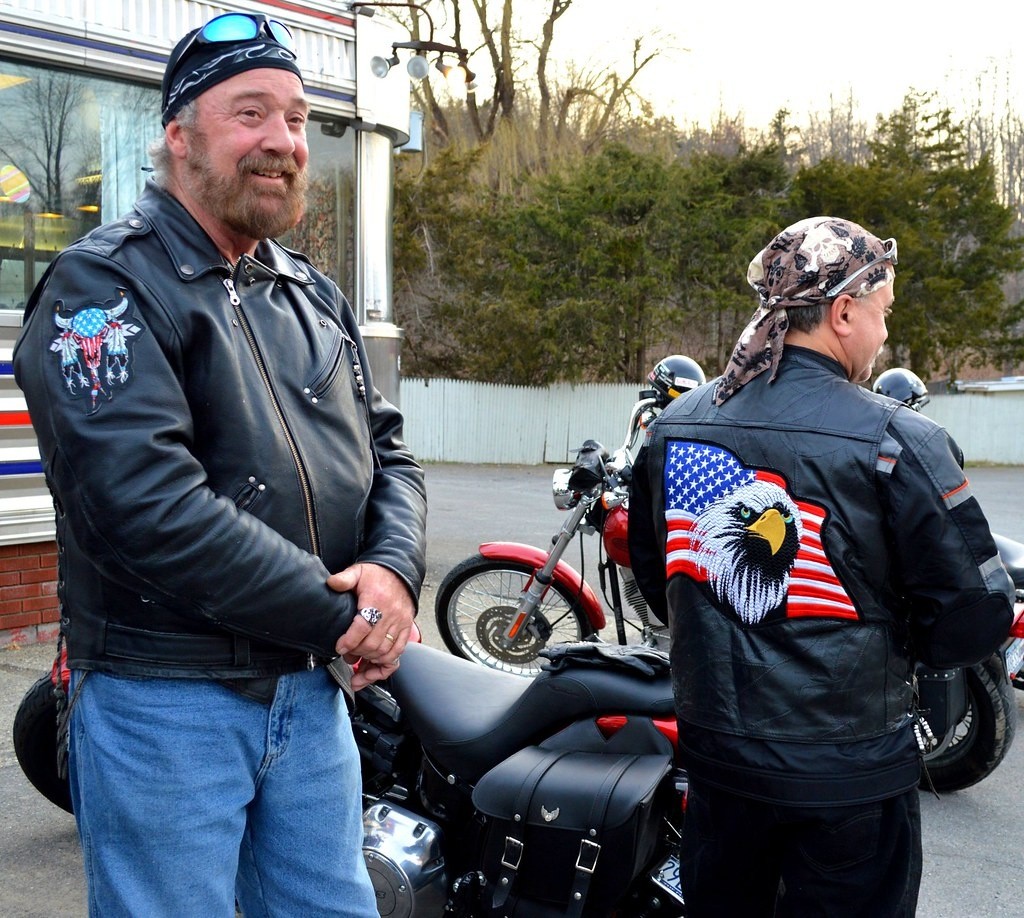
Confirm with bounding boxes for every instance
[539,639,670,683]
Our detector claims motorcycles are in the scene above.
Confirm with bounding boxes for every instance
[435,387,1024,797]
[13,637,686,917]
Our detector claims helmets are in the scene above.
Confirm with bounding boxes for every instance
[647,355,706,409]
[873,368,928,408]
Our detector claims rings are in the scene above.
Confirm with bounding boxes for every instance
[385,633,395,644]
[356,606,382,629]
[392,657,400,666]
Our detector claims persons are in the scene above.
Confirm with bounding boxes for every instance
[628,216,1016,918]
[11,12,427,918]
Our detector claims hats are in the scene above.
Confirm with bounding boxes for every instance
[160,26,305,130]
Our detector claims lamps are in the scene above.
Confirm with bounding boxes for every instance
[349,2,476,83]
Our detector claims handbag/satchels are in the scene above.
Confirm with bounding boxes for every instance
[468,744,673,917]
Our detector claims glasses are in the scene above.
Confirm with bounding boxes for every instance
[161,13,297,115]
[826,238,898,295]
